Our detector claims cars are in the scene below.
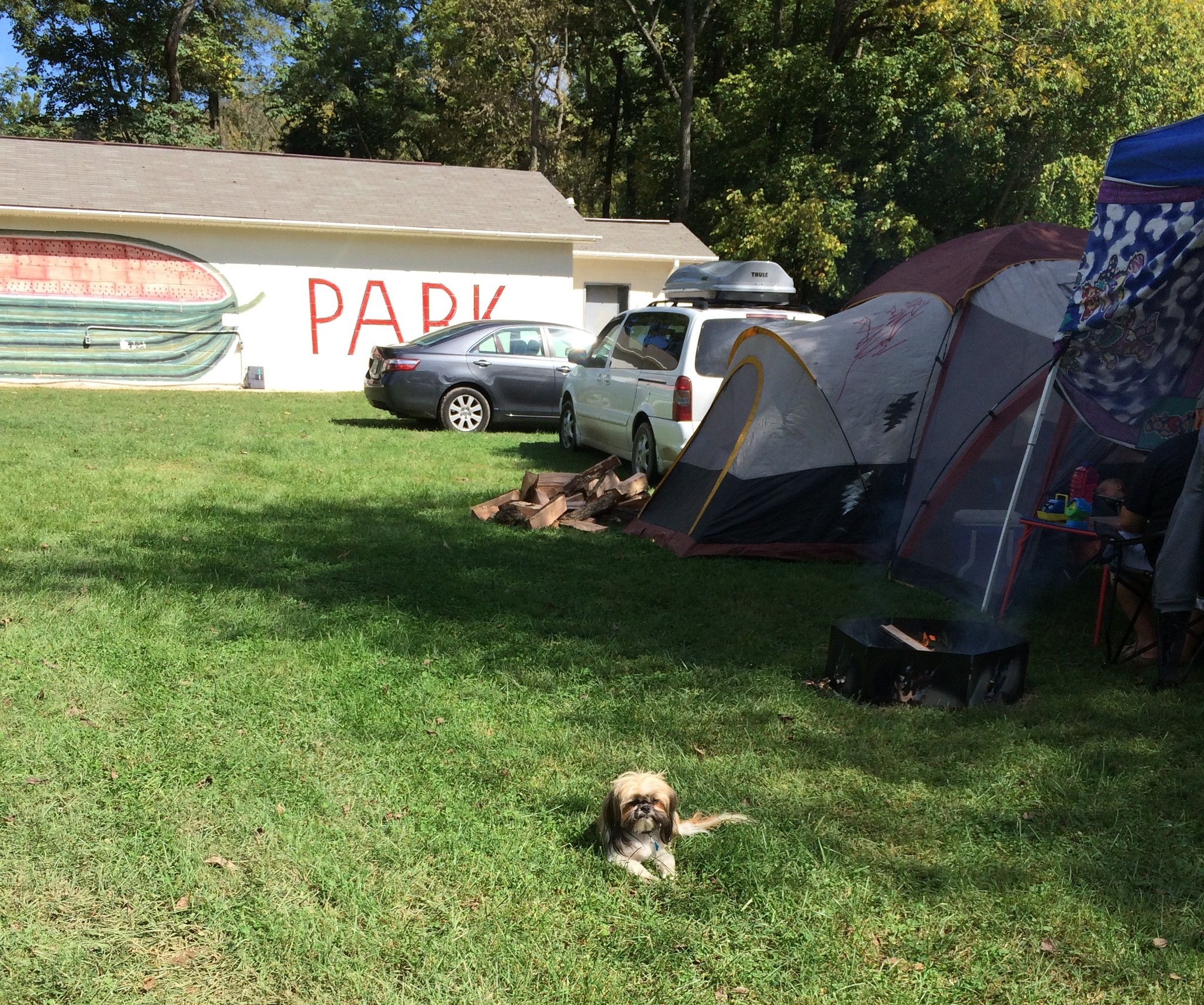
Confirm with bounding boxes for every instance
[364,317,597,432]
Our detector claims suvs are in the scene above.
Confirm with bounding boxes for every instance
[556,260,828,492]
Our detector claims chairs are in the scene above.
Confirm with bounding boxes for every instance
[513,340,539,356]
[1093,518,1204,692]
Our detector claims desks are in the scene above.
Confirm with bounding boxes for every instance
[998,512,1109,645]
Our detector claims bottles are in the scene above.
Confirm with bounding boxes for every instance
[1068,462,1099,525]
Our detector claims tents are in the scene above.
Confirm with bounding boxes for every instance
[623,220,1091,604]
[980,113,1203,636]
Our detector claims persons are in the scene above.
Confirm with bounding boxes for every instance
[1118,392,1204,664]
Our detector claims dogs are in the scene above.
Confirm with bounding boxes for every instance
[578,764,760,882]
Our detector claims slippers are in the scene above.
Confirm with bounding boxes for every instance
[1122,643,1158,665]
